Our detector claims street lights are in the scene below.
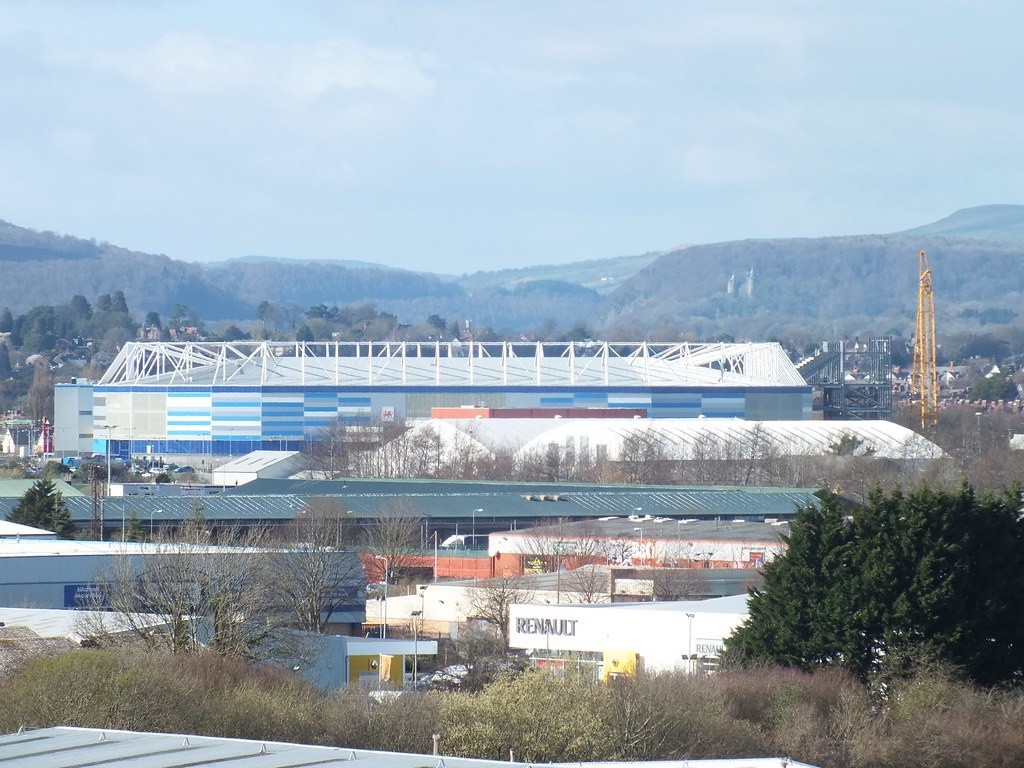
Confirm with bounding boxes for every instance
[229,428,234,456]
[376,595,385,639]
[375,555,387,639]
[337,511,353,546]
[411,611,421,690]
[696,550,713,568]
[682,654,697,674]
[634,528,642,565]
[151,510,163,540]
[632,508,642,515]
[105,425,116,497]
[473,509,483,544]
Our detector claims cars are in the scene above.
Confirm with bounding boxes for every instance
[174,466,194,473]
